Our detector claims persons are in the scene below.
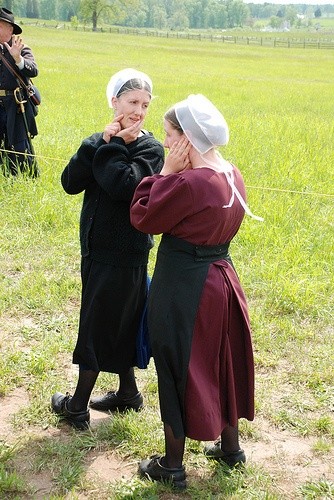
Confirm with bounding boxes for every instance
[128,91,264,485]
[51,68,165,430]
[0,7,41,179]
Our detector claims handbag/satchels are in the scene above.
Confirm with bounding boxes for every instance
[27,80,41,105]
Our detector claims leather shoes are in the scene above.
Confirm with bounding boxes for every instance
[203,441,245,469]
[52,391,90,430]
[90,390,144,413]
[138,456,186,489]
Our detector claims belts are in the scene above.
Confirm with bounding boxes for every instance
[0,90,19,97]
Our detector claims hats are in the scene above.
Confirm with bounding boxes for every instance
[0,7,22,34]
[106,68,153,108]
[174,93,229,155]
